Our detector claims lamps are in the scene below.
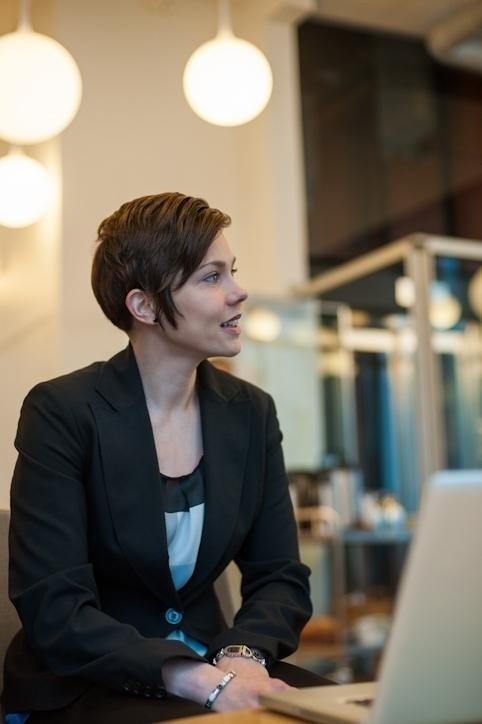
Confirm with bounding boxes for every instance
[182,0,275,132]
[0,146,59,232]
[1,0,90,140]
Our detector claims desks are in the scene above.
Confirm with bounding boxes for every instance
[297,529,416,653]
[150,706,308,724]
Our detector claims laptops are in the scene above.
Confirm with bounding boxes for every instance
[257,468,482,724]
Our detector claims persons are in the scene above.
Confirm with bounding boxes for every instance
[0,191,339,723]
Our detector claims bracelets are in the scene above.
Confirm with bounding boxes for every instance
[204,670,237,707]
[213,645,267,668]
